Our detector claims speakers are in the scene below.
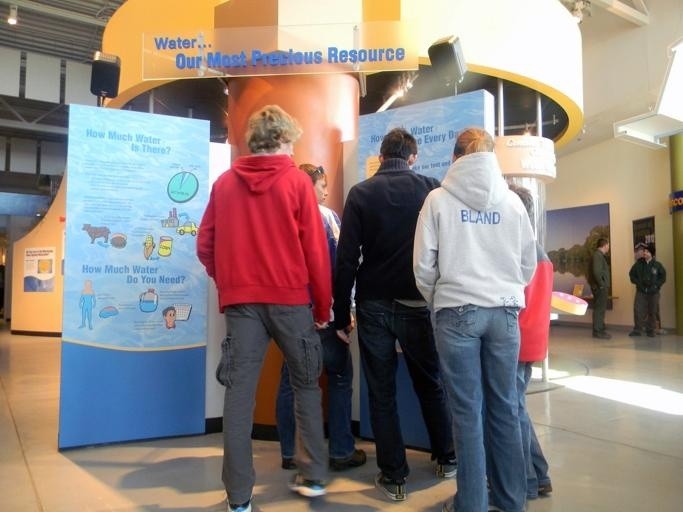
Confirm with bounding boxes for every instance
[90,50,121,98]
[427,34,467,90]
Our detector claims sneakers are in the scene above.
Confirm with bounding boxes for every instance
[591,326,614,343]
[373,468,410,501]
[226,493,255,512]
[485,491,528,512]
[442,493,461,512]
[646,329,659,338]
[285,471,327,499]
[432,455,460,479]
[328,447,368,473]
[280,452,299,471]
[538,478,556,498]
[629,329,643,338]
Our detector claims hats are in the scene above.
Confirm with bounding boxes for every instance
[644,245,656,258]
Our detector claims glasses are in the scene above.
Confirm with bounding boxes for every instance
[307,164,327,177]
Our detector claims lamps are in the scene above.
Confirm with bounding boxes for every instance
[6,3,18,26]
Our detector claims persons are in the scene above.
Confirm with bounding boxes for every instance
[587,239,612,339]
[412,128,537,512]
[331,129,459,501]
[629,247,666,337]
[275,164,366,472]
[509,185,553,499]
[196,105,334,512]
